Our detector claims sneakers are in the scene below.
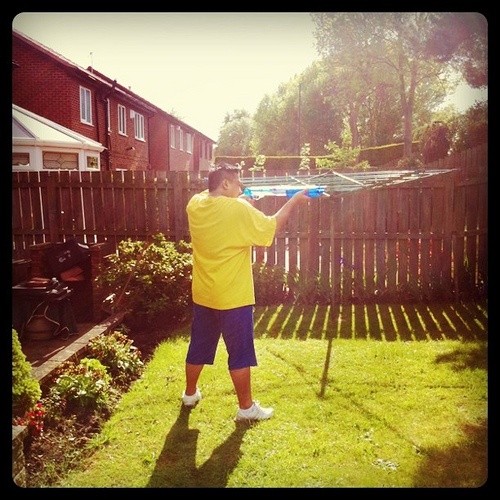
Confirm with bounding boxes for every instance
[236,400,274,422]
[182,388,202,406]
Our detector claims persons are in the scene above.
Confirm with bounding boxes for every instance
[181,162,312,420]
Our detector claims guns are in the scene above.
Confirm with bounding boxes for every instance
[243,184,327,201]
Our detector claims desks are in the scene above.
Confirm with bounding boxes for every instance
[13,277,78,343]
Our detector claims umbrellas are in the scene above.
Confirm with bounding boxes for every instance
[12,102,108,172]
